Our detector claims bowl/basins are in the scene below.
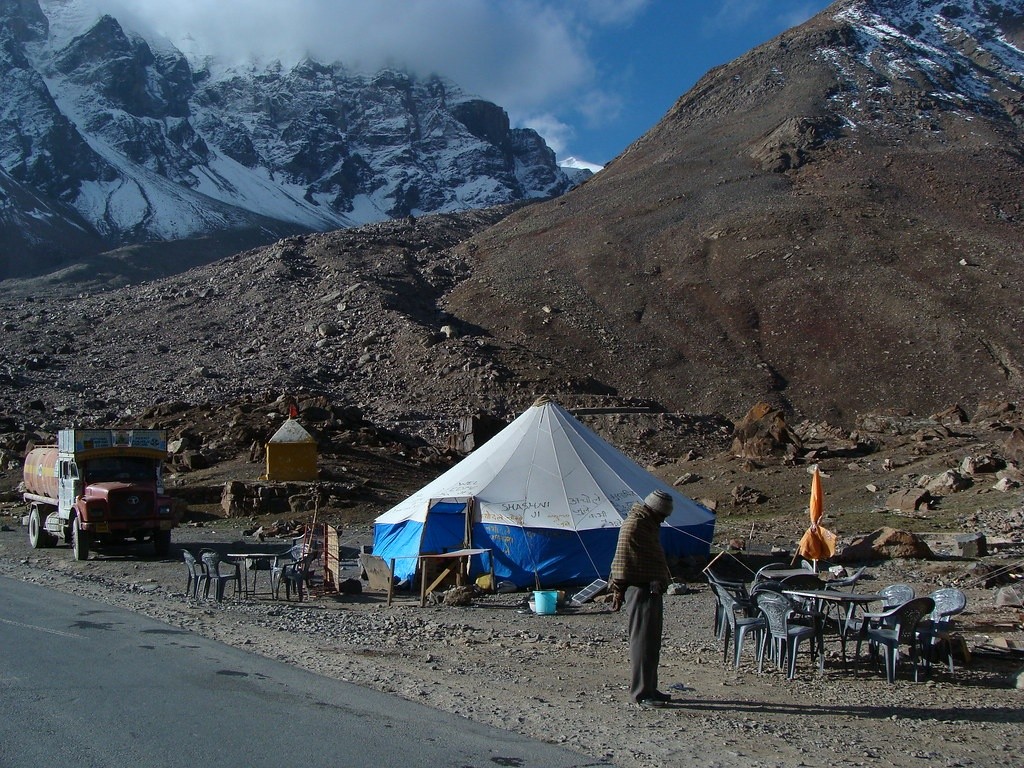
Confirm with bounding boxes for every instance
[528,600,536,613]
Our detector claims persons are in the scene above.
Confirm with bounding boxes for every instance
[607,489,675,708]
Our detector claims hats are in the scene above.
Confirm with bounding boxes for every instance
[644,489,674,516]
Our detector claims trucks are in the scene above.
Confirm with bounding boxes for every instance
[22,427,177,562]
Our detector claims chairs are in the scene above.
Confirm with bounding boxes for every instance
[195,547,241,602]
[271,543,313,599]
[180,546,218,599]
[702,568,966,684]
[275,551,318,603]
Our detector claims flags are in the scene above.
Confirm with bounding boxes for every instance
[289,403,297,417]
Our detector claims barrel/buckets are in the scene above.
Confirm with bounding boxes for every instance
[532,589,558,615]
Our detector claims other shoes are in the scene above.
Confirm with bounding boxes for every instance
[633,689,671,707]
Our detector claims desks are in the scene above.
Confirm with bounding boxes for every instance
[228,553,279,605]
[388,548,496,608]
[758,569,819,581]
[781,589,889,677]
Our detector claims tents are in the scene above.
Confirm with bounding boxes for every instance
[371,393,716,587]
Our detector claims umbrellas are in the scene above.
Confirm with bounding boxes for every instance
[798,465,837,575]
